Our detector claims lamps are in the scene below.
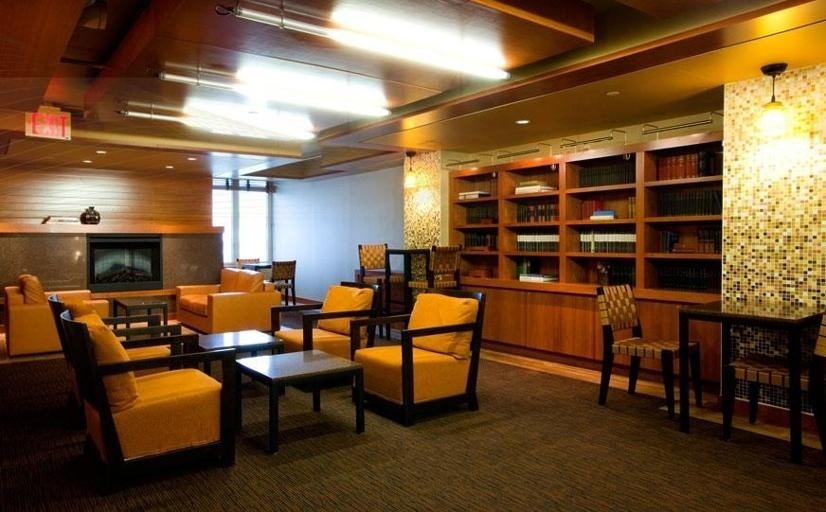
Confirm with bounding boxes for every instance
[753,62,793,139]
[402,151,419,189]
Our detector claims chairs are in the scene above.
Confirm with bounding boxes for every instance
[60,308,236,492]
[236,257,260,268]
[355,243,405,314]
[351,286,487,427]
[406,242,463,310]
[595,282,702,416]
[271,280,382,351]
[47,293,200,433]
[722,348,826,466]
[262,260,296,306]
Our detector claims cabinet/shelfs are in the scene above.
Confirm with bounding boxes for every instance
[448,129,723,386]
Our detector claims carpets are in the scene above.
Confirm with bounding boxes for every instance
[61,357,825,511]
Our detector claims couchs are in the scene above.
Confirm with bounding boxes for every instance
[4,274,110,357]
[174,267,282,333]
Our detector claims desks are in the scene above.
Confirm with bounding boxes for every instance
[198,329,286,397]
[112,296,169,341]
[234,349,365,455]
[675,296,826,464]
[384,246,430,339]
[241,263,272,271]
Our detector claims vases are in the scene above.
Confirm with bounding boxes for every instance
[79,204,100,225]
